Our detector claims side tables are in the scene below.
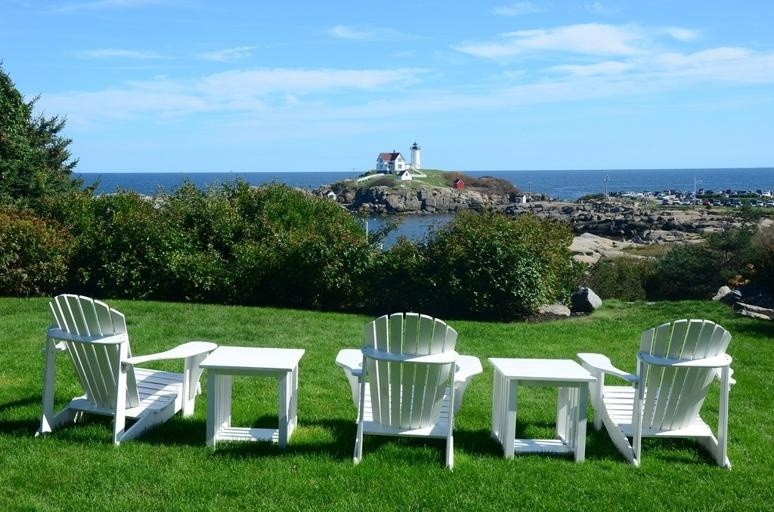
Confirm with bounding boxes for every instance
[487,357,598,463]
[198,346,305,450]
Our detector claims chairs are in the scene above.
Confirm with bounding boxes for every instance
[334,312,482,471]
[576,319,736,469]
[35,293,217,447]
